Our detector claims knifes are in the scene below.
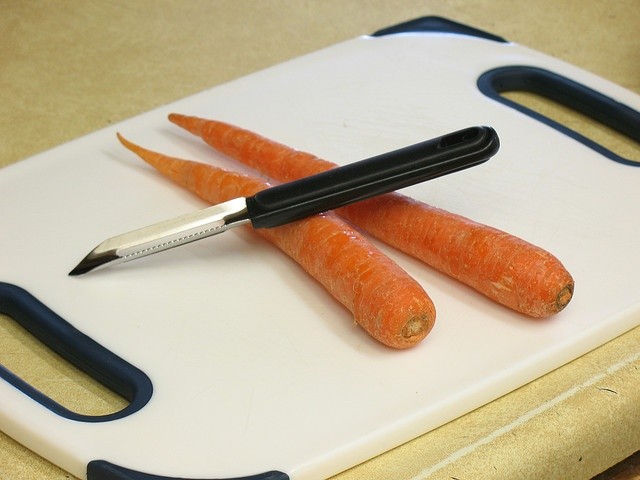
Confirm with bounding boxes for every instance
[66,122,501,278]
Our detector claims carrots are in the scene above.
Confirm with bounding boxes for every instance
[116,130,436,350]
[167,112,576,319]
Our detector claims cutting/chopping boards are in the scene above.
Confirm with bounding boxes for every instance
[0,15,639,480]
